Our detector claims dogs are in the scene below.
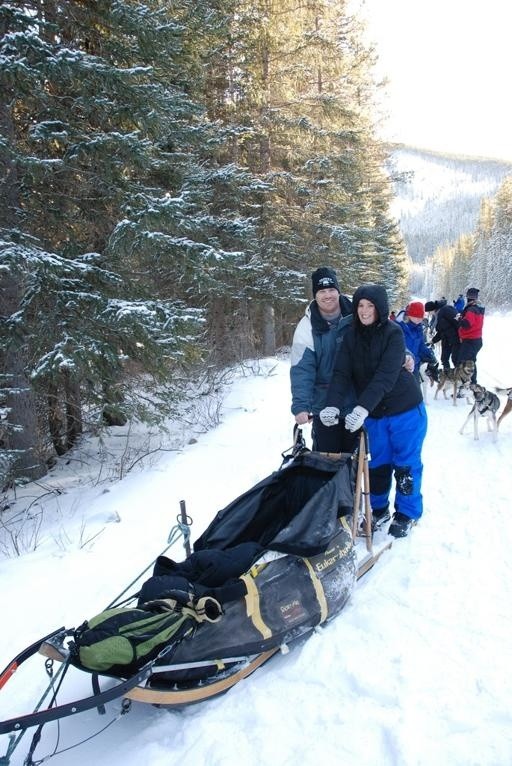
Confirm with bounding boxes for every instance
[433,358,477,406]
[495,381,512,434]
[418,361,442,404]
[456,382,501,448]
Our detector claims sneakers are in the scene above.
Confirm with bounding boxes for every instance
[389,512,417,537]
[360,501,390,531]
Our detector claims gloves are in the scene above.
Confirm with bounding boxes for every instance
[345,405,369,433]
[319,407,340,427]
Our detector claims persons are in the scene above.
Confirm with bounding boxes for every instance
[320,286,427,538]
[390,289,484,399]
[290,266,359,458]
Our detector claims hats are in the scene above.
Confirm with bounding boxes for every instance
[467,288,479,299]
[425,301,437,312]
[405,302,423,319]
[312,268,340,299]
